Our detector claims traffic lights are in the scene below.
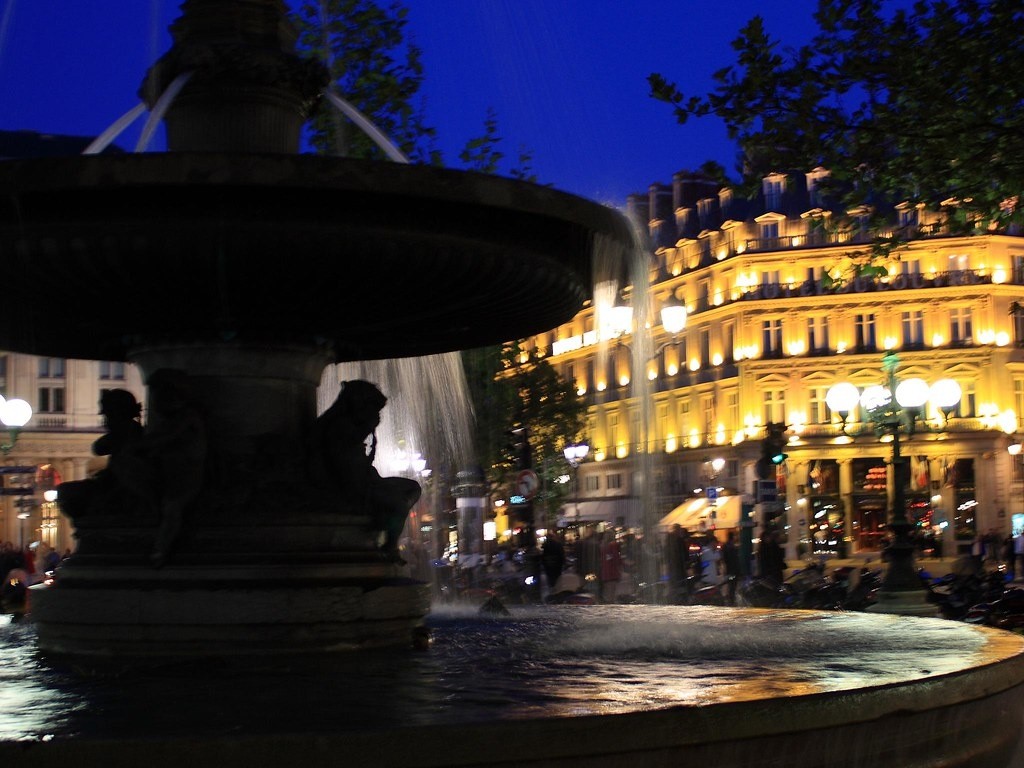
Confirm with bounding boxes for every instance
[761,435,790,468]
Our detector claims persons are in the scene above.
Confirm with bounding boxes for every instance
[0,542,71,612]
[311,379,422,567]
[412,518,1024,612]
[57,367,207,571]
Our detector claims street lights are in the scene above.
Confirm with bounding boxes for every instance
[825,376,962,619]
[564,441,591,573]
[607,294,686,605]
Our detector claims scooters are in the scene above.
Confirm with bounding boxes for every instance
[632,551,1023,630]
